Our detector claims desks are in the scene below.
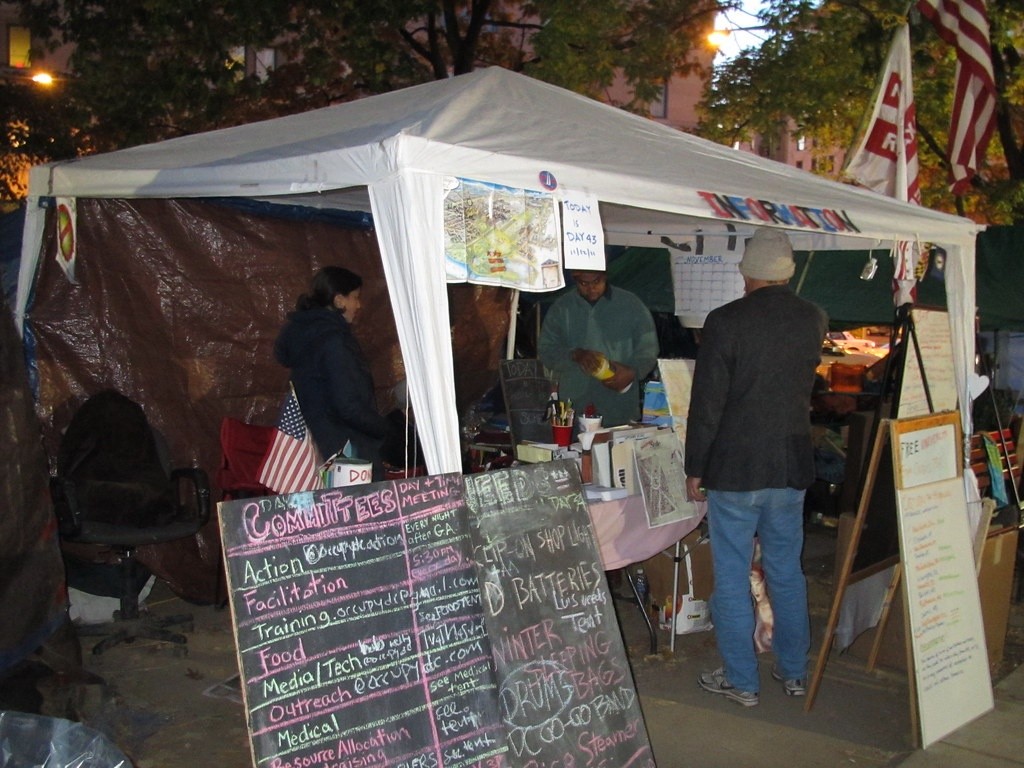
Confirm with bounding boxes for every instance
[584,490,709,656]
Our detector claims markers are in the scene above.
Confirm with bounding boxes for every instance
[551,393,560,426]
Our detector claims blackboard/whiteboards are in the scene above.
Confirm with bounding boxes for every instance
[840,410,995,750]
[216,471,510,768]
[462,458,658,768]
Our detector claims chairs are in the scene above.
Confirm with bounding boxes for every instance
[49,390,210,654]
[215,416,274,527]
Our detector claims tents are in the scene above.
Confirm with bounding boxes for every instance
[16,63,982,505]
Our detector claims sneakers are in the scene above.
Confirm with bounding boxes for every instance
[771,665,806,695]
[698,667,759,707]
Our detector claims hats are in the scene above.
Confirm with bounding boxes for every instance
[737,227,796,281]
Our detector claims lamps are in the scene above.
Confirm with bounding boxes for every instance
[860,250,878,281]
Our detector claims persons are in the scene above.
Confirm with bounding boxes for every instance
[682,228,829,705]
[537,270,659,595]
[273,264,395,482]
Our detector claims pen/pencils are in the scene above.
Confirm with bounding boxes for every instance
[552,397,574,426]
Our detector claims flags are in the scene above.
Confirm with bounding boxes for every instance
[914,0,997,194]
[256,388,324,494]
[848,20,920,305]
